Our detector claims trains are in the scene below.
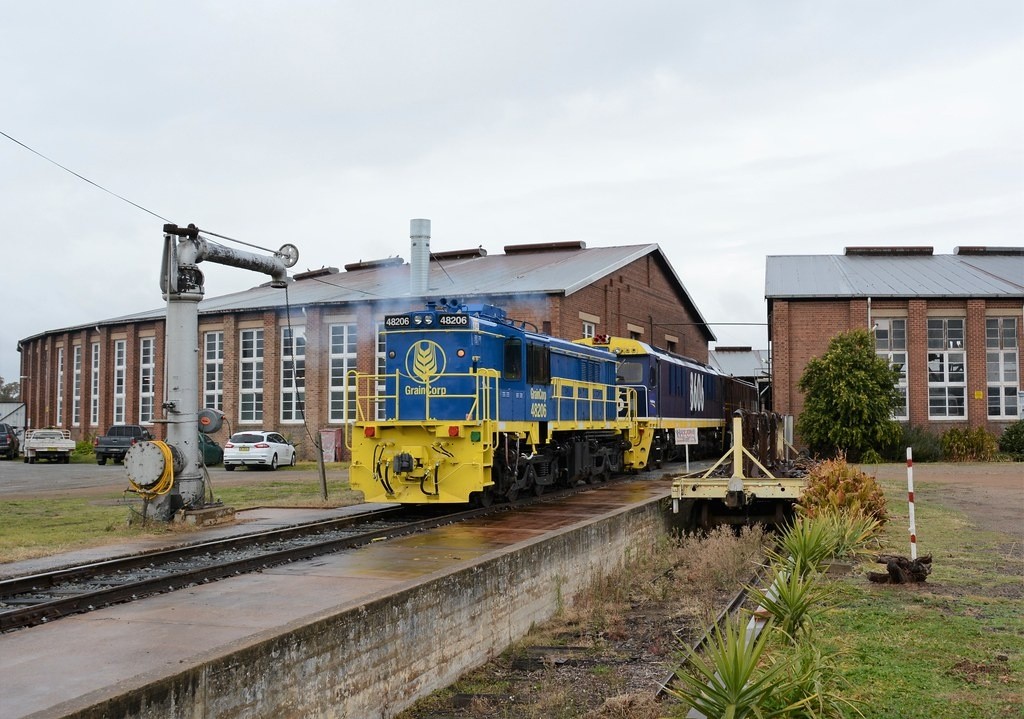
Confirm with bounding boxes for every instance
[343,297,759,508]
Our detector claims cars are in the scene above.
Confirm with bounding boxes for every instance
[223,430,297,471]
[163,432,224,465]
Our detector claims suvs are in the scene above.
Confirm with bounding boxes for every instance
[0,423,19,460]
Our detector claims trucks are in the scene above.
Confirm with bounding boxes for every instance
[23,429,75,464]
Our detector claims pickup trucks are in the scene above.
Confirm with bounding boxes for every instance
[94,425,156,465]
